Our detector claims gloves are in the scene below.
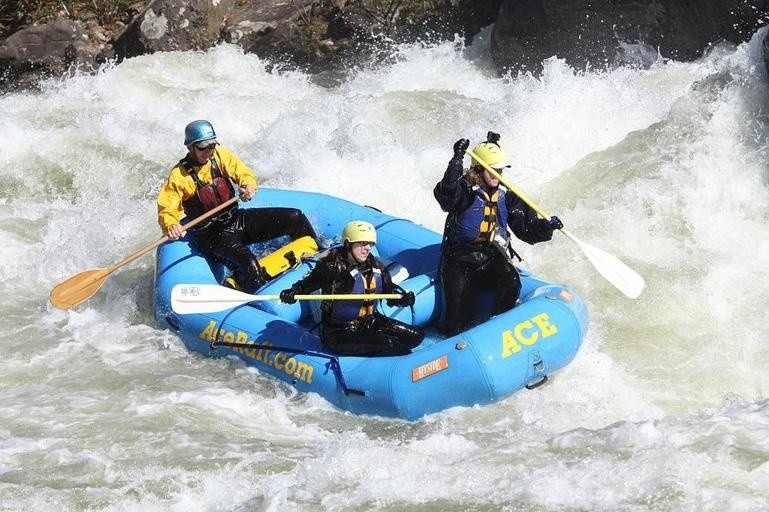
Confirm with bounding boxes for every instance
[453,138,470,158]
[392,291,416,307]
[279,285,301,305]
[541,215,564,233]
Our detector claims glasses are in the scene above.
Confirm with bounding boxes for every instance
[352,241,375,247]
[194,144,217,151]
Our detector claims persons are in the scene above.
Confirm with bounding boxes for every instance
[434,138,564,322]
[279,220,423,356]
[158,119,319,292]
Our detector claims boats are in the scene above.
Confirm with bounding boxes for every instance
[152,186,589,422]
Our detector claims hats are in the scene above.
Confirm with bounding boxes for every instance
[195,139,221,149]
[489,161,512,171]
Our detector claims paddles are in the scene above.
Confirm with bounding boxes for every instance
[466,148,647,299]
[171,284,404,316]
[49,193,241,309]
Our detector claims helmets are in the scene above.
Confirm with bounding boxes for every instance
[342,220,378,246]
[183,119,217,146]
[470,139,512,170]
